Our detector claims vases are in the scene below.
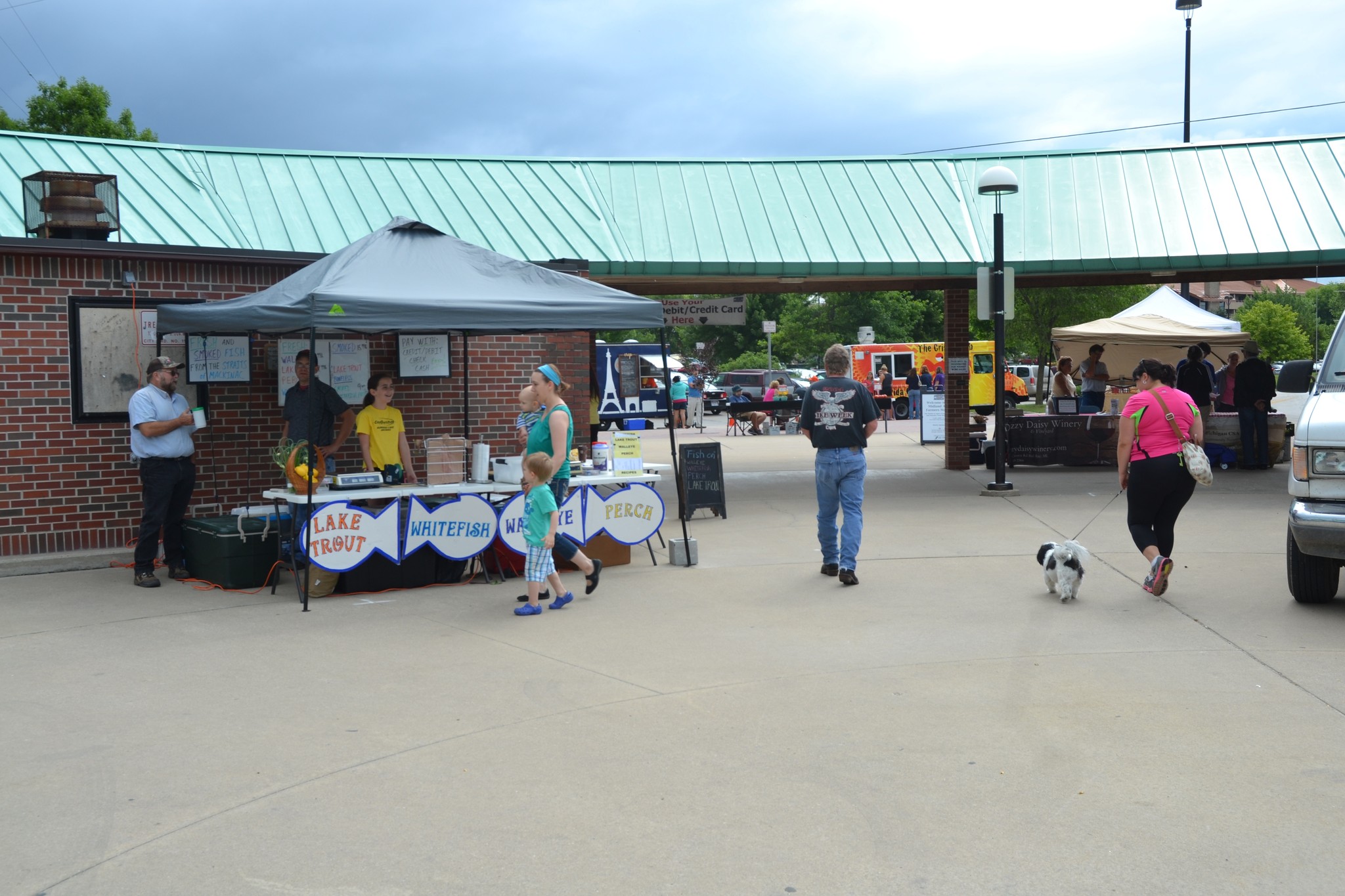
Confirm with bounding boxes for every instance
[490,455,524,485]
[284,471,296,493]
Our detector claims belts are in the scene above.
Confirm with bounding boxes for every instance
[150,456,184,461]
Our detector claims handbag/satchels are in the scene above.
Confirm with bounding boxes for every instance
[917,374,922,387]
[1181,441,1213,485]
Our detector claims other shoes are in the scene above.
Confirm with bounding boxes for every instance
[1259,463,1268,469]
[586,558,602,594]
[820,562,839,576]
[888,418,894,420]
[696,425,706,428]
[839,568,859,584]
[748,429,758,436]
[685,425,690,429]
[517,587,550,601]
[878,418,883,421]
[756,430,763,435]
[1241,464,1255,470]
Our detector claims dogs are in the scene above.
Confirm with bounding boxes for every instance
[1037,540,1089,603]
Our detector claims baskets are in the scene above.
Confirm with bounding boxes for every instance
[284,443,326,495]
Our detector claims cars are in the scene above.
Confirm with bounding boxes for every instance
[676,357,710,379]
[1270,304,1345,608]
[710,368,828,409]
[649,371,729,415]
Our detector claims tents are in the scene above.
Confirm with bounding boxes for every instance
[153,215,688,616]
[1045,286,1251,412]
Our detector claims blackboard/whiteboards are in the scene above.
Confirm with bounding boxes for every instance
[184,333,252,386]
[679,442,726,508]
[619,354,639,397]
[276,338,370,411]
[396,331,451,380]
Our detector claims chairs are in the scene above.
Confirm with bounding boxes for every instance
[774,394,802,434]
[727,411,753,436]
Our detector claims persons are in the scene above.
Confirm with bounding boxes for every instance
[517,385,547,456]
[799,343,893,585]
[646,377,658,388]
[1050,344,1111,466]
[687,368,707,428]
[518,364,601,601]
[280,349,357,570]
[641,377,648,388]
[905,366,945,420]
[129,356,197,588]
[1116,358,1204,597]
[670,376,689,428]
[513,452,574,615]
[764,377,785,426]
[355,374,417,505]
[728,385,766,435]
[1176,340,1277,470]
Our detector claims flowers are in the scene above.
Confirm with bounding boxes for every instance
[271,435,308,479]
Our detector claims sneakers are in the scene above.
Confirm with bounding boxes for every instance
[1143,556,1174,596]
[167,565,189,580]
[134,572,161,587]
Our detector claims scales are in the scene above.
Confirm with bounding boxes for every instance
[325,471,385,491]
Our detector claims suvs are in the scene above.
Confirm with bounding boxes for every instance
[1004,364,1054,400]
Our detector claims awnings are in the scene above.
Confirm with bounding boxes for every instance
[638,354,684,369]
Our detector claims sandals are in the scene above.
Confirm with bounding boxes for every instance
[513,604,542,616]
[549,591,573,609]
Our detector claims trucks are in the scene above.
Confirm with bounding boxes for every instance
[594,339,673,431]
[814,342,945,421]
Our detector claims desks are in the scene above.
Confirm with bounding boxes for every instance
[263,459,672,603]
[990,413,1121,469]
[873,397,896,433]
[726,400,803,437]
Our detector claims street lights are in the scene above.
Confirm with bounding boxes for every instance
[1174,0,1202,143]
[979,166,1020,489]
[1224,296,1233,321]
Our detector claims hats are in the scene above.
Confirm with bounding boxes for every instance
[147,355,186,374]
[1239,340,1263,353]
[296,349,318,368]
[732,385,743,392]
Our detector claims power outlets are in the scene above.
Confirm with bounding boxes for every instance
[122,271,134,287]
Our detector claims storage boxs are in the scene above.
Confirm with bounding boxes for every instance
[785,421,799,436]
[577,535,631,568]
[183,505,292,587]
[762,421,781,435]
[969,433,987,465]
[623,418,646,431]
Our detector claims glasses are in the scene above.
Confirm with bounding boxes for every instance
[296,363,310,369]
[155,370,180,378]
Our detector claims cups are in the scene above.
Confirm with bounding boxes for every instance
[577,445,588,463]
[592,442,609,471]
[192,407,206,429]
